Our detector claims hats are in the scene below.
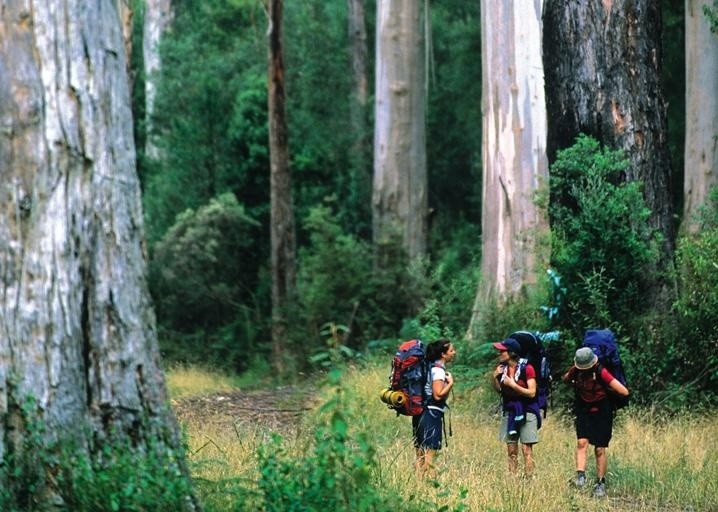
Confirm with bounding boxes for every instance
[573,347,598,371]
[493,338,521,353]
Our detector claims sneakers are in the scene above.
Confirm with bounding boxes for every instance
[568,474,586,491]
[591,481,605,497]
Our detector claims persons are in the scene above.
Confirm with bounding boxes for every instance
[411,338,456,472]
[491,340,538,478]
[562,346,629,498]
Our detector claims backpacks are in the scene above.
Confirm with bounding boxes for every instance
[390,339,446,416]
[498,331,553,409]
[571,329,629,411]
